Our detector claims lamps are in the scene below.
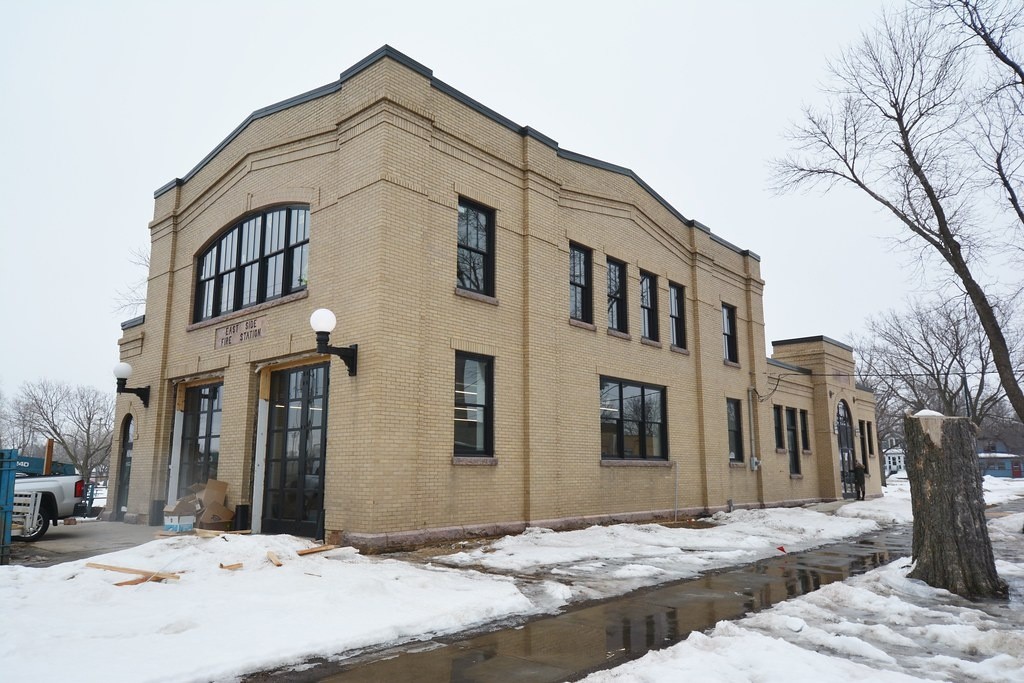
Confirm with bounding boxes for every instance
[115,361,150,409]
[311,307,357,375]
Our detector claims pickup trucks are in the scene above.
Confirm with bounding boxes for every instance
[305,456,320,519]
[11,472,87,543]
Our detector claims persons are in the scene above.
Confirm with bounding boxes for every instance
[854,462,865,500]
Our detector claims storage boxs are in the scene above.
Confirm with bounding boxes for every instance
[163,479,236,533]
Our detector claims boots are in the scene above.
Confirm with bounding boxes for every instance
[861,491,865,500]
[855,491,860,500]
